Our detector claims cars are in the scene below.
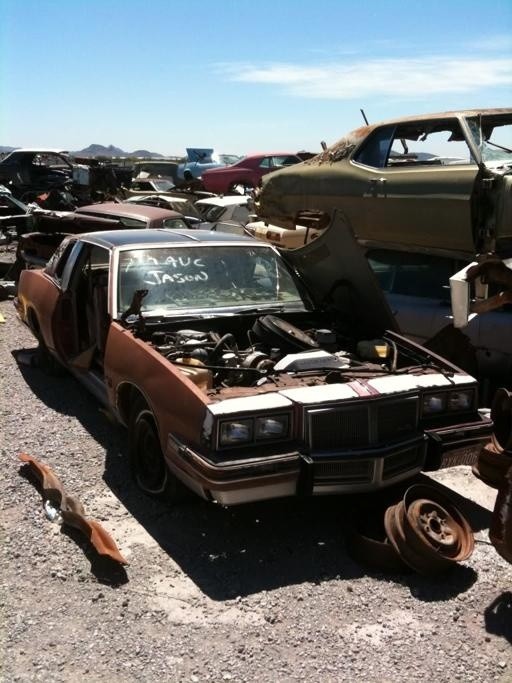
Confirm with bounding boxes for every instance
[12,230,495,510]
[374,262,511,366]
[257,105,512,258]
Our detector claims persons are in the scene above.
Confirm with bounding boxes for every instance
[180,169,205,191]
